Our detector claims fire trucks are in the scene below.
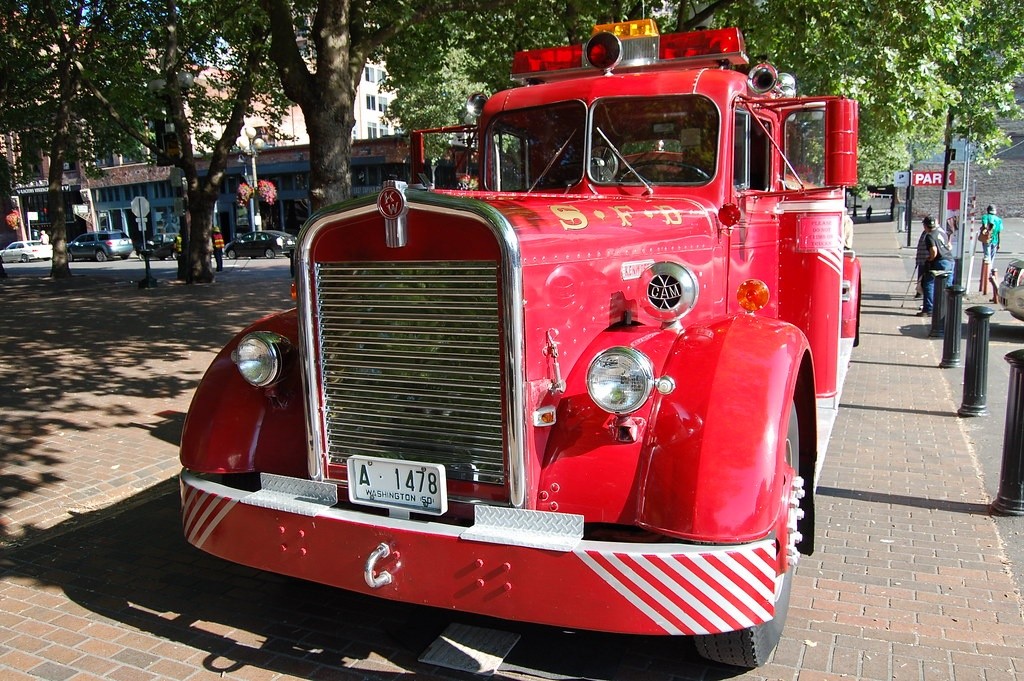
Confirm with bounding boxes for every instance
[178,16,869,673]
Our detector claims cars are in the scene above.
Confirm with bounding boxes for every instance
[222,231,298,259]
[997,255,1024,324]
[135,232,177,261]
[1,241,53,263]
[155,239,179,260]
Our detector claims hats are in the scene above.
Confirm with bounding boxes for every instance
[922,217,936,227]
[988,204,997,214]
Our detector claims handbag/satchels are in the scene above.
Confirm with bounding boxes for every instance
[978,214,994,244]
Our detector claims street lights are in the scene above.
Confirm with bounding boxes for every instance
[236,126,266,233]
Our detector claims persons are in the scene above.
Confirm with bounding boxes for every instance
[211,226,224,272]
[915,214,956,317]
[39,230,49,245]
[866,205,872,219]
[982,204,1004,279]
[174,229,183,257]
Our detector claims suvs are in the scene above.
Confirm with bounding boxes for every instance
[67,230,134,261]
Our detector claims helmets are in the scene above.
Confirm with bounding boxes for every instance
[213,226,221,232]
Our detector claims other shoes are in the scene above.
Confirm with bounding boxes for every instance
[917,312,932,317]
[912,294,924,300]
